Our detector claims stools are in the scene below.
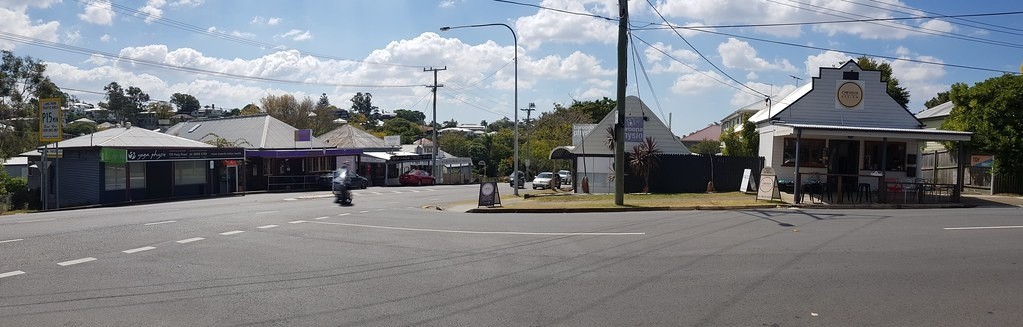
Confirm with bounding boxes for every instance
[817,183,834,203]
[856,183,873,204]
[801,183,814,203]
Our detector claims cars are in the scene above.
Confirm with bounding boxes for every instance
[321,172,368,189]
[510,171,525,188]
[533,172,561,189]
[558,170,571,185]
[399,169,435,185]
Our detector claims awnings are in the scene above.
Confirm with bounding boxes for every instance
[360,152,432,163]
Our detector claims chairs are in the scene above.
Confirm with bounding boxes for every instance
[885,177,949,203]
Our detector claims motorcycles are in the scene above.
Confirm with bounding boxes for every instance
[332,167,353,207]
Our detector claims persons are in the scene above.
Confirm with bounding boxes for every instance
[340,160,350,206]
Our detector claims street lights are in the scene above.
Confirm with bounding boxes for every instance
[440,23,519,196]
